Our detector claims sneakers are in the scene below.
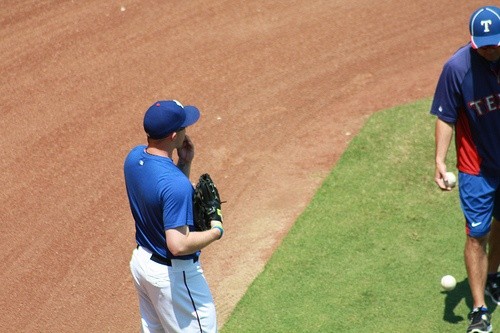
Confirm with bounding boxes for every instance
[466,304,493,333]
[485,272,500,305]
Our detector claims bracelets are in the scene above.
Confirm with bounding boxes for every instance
[213,225,223,240]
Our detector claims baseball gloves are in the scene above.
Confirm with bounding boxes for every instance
[193,173,222,232]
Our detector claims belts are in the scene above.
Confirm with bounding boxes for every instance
[136,244,199,267]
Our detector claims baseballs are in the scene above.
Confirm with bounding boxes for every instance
[441,275,456,291]
[443,172,457,187]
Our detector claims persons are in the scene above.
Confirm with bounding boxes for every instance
[124,100,225,333]
[429,6,500,333]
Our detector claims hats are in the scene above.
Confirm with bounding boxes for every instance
[143,100,200,140]
[469,6,500,49]
